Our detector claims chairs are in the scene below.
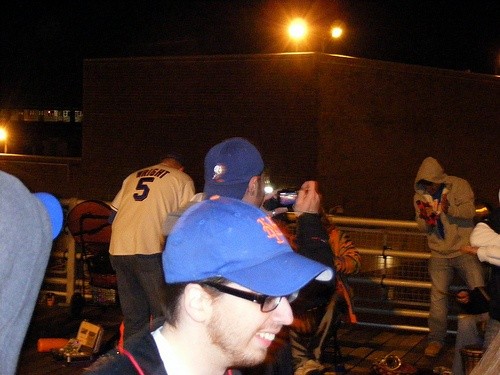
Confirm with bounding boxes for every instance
[68,199,122,314]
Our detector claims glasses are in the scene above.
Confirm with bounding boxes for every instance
[198,278,300,314]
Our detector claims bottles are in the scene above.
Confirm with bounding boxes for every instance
[46,292,55,308]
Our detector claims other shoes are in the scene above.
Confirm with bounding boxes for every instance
[425,341,446,356]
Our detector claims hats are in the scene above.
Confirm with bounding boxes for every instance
[161,197,334,297]
[203,136,264,202]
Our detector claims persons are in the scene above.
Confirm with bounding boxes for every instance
[411,155,494,359]
[106,149,196,348]
[0,168,53,375]
[81,195,333,375]
[257,209,361,375]
[167,137,336,314]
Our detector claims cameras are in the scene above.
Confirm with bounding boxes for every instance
[277,190,298,208]
